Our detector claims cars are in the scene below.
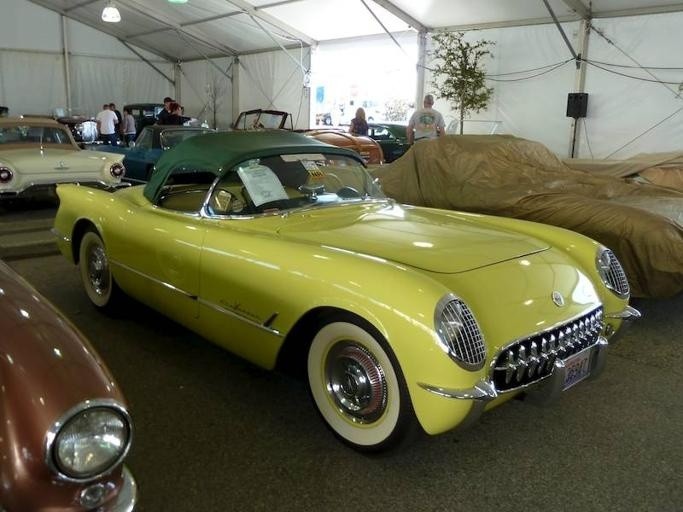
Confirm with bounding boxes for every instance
[98,101,415,185]
[0,259,140,512]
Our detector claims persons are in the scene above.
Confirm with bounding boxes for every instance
[348,108,369,136]
[96,104,119,147]
[123,107,136,147]
[109,103,123,142]
[407,94,446,144]
[161,102,191,126]
[159,97,176,125]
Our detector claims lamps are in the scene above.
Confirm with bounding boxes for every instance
[101,0,121,22]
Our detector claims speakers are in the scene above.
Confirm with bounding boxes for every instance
[566,93,588,118]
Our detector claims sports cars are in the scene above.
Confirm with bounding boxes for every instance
[0,114,128,205]
[48,129,641,455]
